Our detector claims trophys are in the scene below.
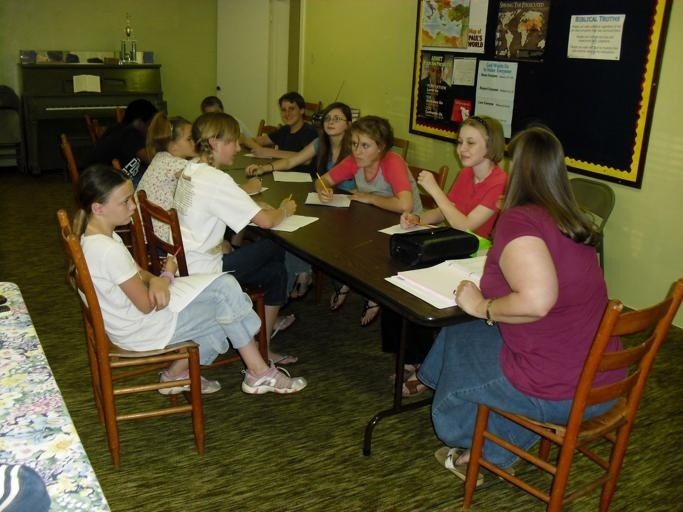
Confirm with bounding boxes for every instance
[120,12,137,62]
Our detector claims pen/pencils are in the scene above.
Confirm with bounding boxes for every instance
[160,247,181,279]
[316,172,329,194]
[289,193,293,201]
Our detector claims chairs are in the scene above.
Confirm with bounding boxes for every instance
[60,132,141,266]
[56,209,206,469]
[137,187,271,405]
[310,137,409,305]
[84,113,109,169]
[568,176,615,278]
[256,117,277,141]
[294,97,323,123]
[115,104,124,126]
[463,278,683,512]
[408,161,450,228]
[0,83,29,176]
[111,156,149,272]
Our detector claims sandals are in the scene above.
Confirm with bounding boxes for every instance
[392,363,429,398]
[272,314,295,330]
[361,299,380,326]
[291,273,313,298]
[329,285,350,311]
[268,353,300,366]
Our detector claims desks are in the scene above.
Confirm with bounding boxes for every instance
[184,143,469,457]
[0,279,112,511]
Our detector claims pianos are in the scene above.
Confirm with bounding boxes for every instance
[18,63,167,177]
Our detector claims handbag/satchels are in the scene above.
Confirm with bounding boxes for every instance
[389,227,479,271]
[0,464,51,512]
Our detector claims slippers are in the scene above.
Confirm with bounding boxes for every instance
[434,447,485,487]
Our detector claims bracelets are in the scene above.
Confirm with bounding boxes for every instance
[416,215,421,225]
[159,270,174,285]
[486,297,495,327]
[267,161,274,171]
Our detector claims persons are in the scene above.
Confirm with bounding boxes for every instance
[78,98,159,189]
[244,92,321,169]
[414,127,628,487]
[134,110,197,259]
[417,62,455,122]
[200,96,253,145]
[387,115,509,398]
[244,102,356,300]
[314,115,423,328]
[74,164,308,396]
[167,112,300,366]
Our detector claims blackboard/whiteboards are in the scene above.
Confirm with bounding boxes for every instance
[408,1,672,190]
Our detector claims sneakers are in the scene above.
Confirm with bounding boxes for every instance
[241,359,308,395]
[158,369,222,395]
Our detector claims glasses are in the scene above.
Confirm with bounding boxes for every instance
[324,115,348,122]
